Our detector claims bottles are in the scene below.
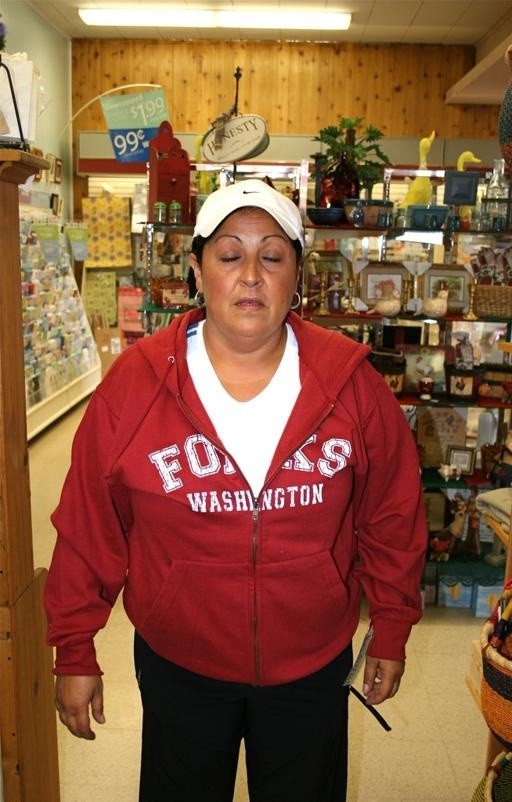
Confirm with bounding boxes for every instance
[153,202,167,226]
[169,203,183,225]
[483,158,510,230]
[353,201,365,228]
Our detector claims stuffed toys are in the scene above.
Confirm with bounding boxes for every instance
[441,150,481,230]
[397,130,436,210]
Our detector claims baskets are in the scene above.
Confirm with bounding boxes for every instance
[473,283,512,319]
[470,602,512,802]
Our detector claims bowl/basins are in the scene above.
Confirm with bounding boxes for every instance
[343,199,394,228]
[305,208,345,224]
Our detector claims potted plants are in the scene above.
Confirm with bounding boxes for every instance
[310,115,395,227]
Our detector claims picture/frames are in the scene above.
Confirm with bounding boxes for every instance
[406,203,449,230]
[311,251,479,478]
[442,170,480,206]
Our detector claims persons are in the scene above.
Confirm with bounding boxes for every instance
[42,177,428,802]
[381,279,395,300]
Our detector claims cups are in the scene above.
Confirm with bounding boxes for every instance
[376,205,507,235]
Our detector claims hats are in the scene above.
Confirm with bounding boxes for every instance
[191,178,305,256]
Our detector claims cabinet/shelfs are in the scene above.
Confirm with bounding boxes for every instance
[137,223,511,566]
